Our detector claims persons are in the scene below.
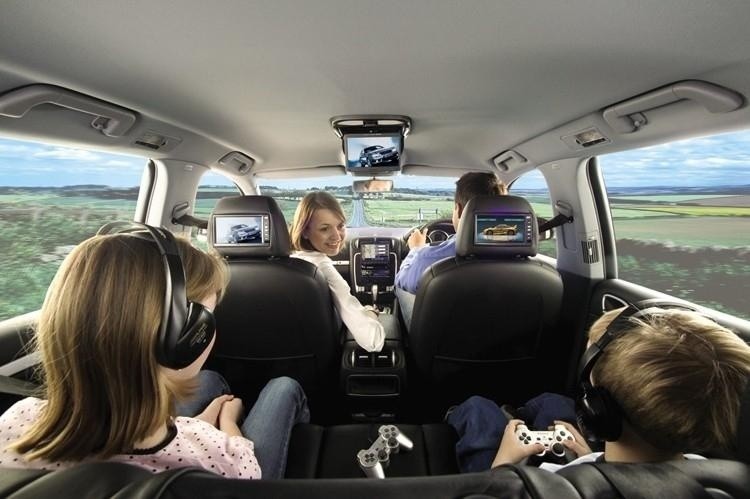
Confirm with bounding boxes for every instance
[396,172,508,332]
[438,297,750,474]
[0,225,310,481]
[289,190,386,353]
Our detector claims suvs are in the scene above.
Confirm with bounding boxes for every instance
[357,144,399,167]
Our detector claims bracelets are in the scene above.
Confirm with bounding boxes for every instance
[366,308,380,316]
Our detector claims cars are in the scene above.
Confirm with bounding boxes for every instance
[483,223,520,236]
[226,223,262,244]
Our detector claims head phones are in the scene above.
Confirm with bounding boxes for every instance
[575,297,700,442]
[96,219,218,370]
